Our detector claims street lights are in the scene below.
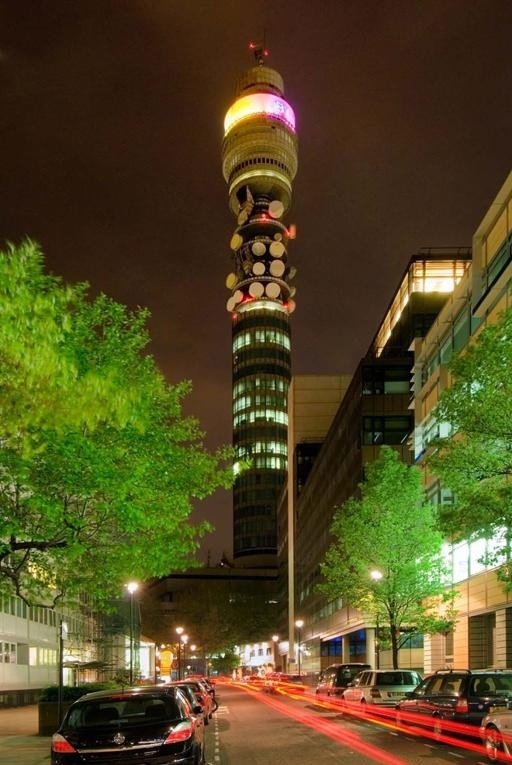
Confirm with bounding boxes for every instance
[372,571,382,669]
[295,619,304,676]
[128,581,139,686]
[154,642,165,683]
[273,635,279,672]
[176,627,197,680]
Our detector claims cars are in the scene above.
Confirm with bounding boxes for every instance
[316,663,371,696]
[51,674,218,765]
[264,671,303,693]
[479,708,512,761]
[341,669,423,707]
[395,670,512,743]
